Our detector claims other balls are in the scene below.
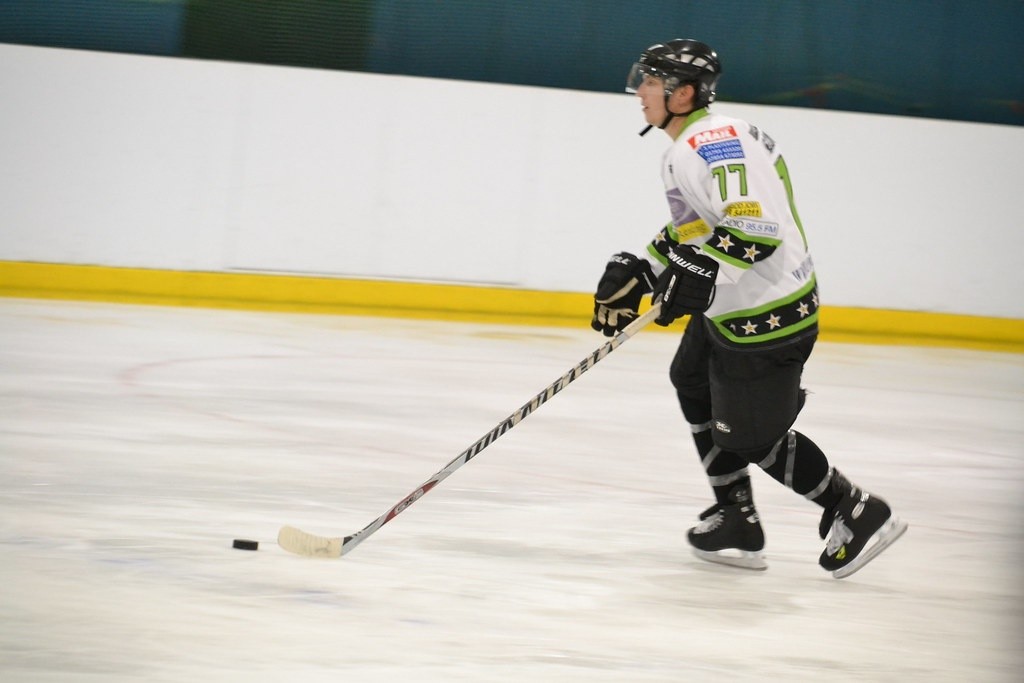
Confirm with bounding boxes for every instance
[232,539,259,550]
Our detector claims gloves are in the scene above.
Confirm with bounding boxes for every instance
[651,245,720,326]
[591,251,655,337]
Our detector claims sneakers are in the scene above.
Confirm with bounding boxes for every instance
[683,476,768,569]
[818,468,907,578]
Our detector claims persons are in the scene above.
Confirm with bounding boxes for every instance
[590,38,910,579]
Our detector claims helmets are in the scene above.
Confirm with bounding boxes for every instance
[636,39,720,103]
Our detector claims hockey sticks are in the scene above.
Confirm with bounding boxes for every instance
[276,300,664,563]
